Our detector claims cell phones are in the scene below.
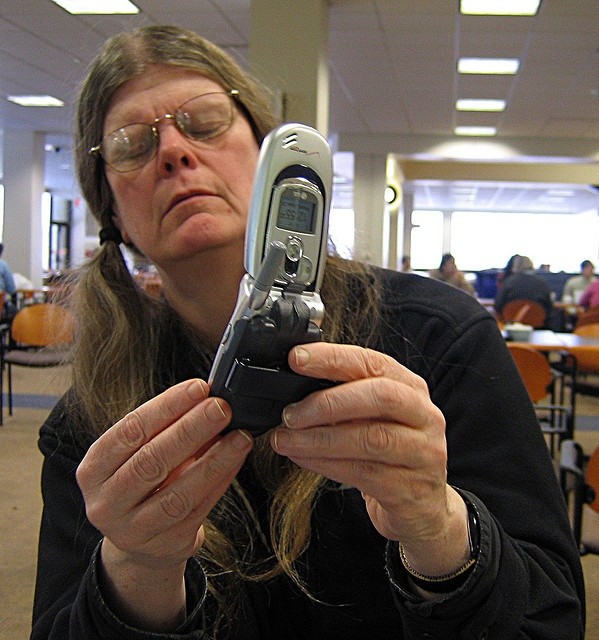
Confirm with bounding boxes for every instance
[205,122,334,439]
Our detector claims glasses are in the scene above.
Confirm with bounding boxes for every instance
[87,88,240,175]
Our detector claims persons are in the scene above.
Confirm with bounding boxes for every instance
[492,255,555,327]
[560,260,598,325]
[399,255,413,273]
[32,24,588,639]
[1,242,17,308]
[430,253,479,298]
[578,278,599,308]
[496,254,521,295]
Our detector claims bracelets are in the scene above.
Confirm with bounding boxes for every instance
[397,496,479,596]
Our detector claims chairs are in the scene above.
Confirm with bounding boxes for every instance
[507,342,559,454]
[575,307,599,325]
[504,301,546,327]
[560,436,599,557]
[4,304,78,414]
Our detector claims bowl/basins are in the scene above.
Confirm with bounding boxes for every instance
[505,325,533,343]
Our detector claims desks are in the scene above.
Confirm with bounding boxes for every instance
[551,302,583,312]
[498,326,599,447]
